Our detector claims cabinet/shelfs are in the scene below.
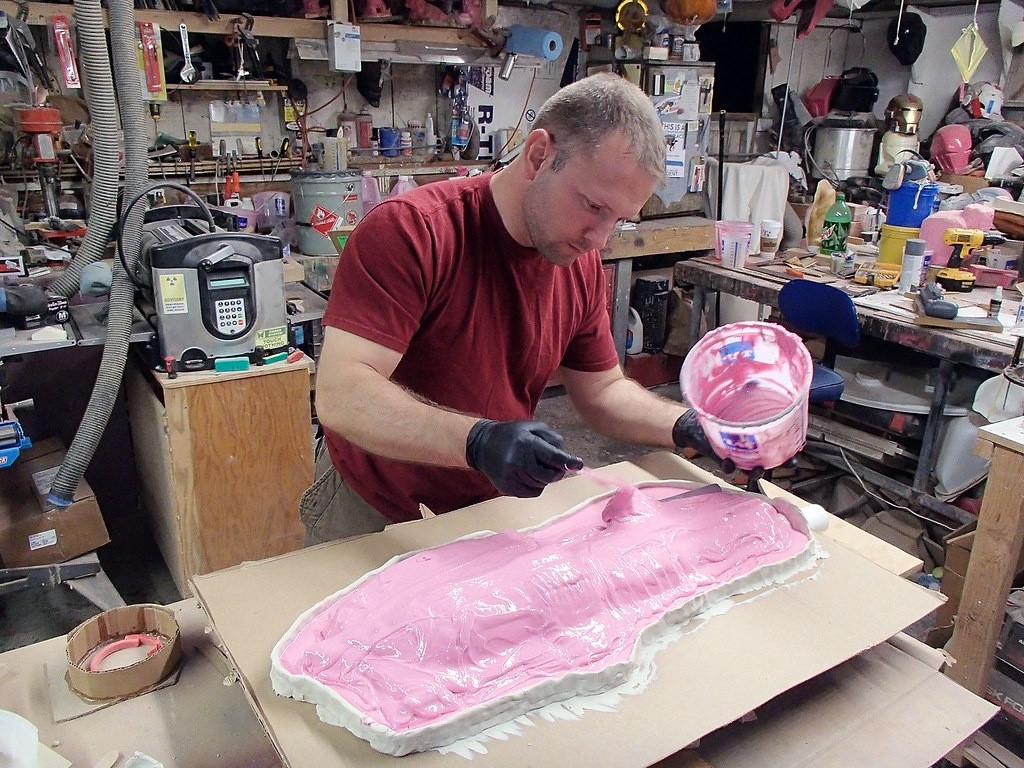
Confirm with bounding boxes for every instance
[0,0,502,90]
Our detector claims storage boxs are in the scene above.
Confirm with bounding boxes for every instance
[326,20,362,73]
[625,351,684,386]
[924,531,1024,741]
[939,174,989,194]
[0,436,112,569]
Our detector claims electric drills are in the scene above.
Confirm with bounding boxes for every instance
[935,228,1007,293]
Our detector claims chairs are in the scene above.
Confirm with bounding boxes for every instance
[731,277,859,492]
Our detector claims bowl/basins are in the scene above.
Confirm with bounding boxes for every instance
[714,220,754,259]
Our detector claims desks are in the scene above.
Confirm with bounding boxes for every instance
[678,260,1024,522]
[605,211,716,372]
[945,415,1024,701]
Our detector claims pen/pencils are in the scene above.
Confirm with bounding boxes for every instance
[786,266,804,278]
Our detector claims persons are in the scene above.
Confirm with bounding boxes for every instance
[298,73,799,548]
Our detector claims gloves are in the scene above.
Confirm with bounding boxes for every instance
[673,409,734,473]
[464,418,583,499]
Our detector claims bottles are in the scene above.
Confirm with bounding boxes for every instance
[224,197,254,210]
[820,194,850,255]
[987,286,1003,319]
[898,238,926,295]
[338,107,437,156]
[1015,296,1024,325]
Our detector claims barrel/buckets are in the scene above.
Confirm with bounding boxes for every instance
[811,127,879,180]
[679,320,814,470]
[289,166,363,256]
[876,223,921,264]
[252,191,289,230]
[886,182,938,228]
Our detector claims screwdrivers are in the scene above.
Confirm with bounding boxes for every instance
[220,139,226,179]
[254,136,269,183]
[187,131,197,184]
[271,138,289,181]
[150,104,167,183]
[231,150,239,194]
[225,152,233,199]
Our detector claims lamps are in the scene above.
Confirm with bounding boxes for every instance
[286,39,542,68]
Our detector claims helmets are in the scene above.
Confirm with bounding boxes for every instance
[929,123,972,173]
[962,81,1003,119]
[884,94,923,135]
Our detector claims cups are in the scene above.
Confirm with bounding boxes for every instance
[604,34,615,49]
[760,219,782,259]
[721,232,750,269]
